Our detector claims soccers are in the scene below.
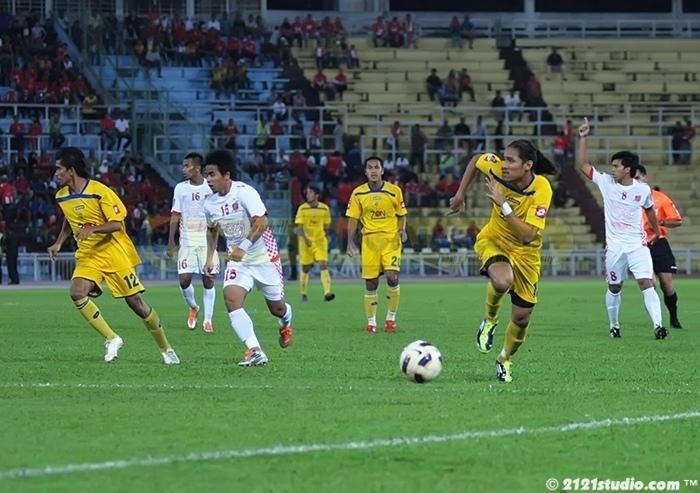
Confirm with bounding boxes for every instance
[400,339,445,384]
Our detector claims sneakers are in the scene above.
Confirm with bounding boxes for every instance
[496,353,513,384]
[664,291,684,329]
[364,325,377,334]
[384,320,396,332]
[653,326,669,339]
[300,293,307,303]
[202,320,213,332]
[237,346,269,366]
[160,348,180,364]
[476,318,499,354]
[279,320,292,348]
[609,327,621,338]
[187,305,199,329]
[324,293,335,301]
[104,333,123,362]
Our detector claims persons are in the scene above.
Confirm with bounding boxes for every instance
[449,139,553,381]
[0,0,578,145]
[294,148,345,184]
[0,151,48,189]
[295,185,335,300]
[576,116,669,340]
[48,146,180,364]
[202,148,293,367]
[166,151,221,333]
[682,120,696,162]
[668,117,684,164]
[0,190,60,285]
[117,190,179,243]
[634,164,683,329]
[346,156,408,332]
